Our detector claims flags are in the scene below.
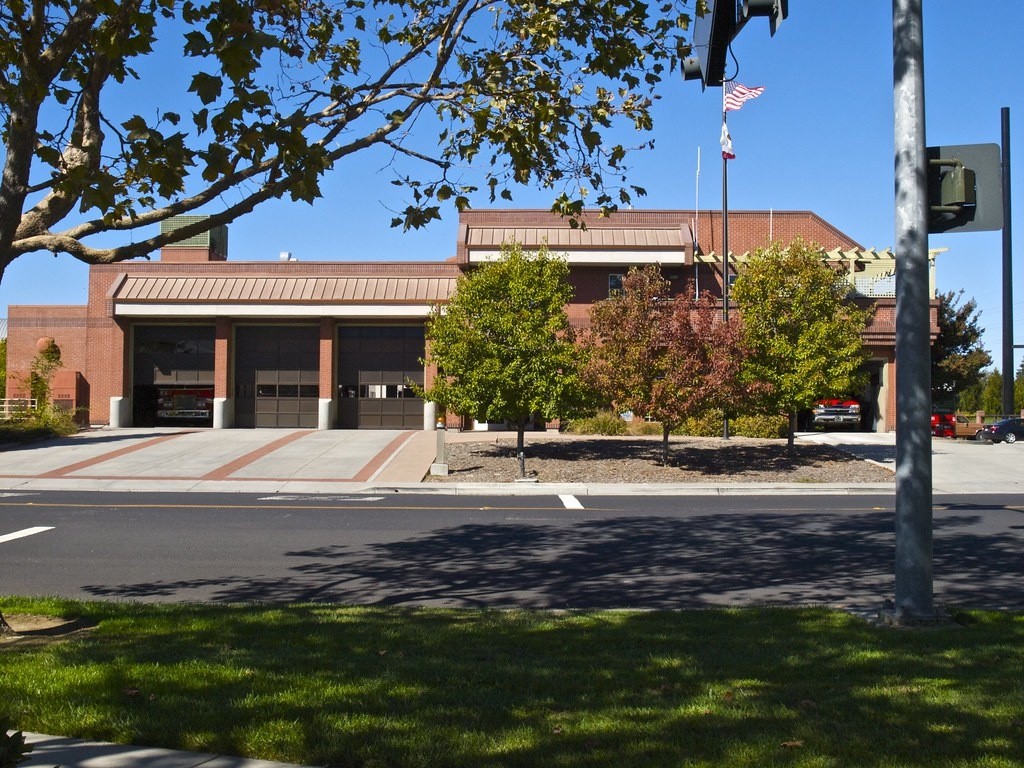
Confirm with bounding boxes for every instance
[723,75,766,113]
[721,118,736,160]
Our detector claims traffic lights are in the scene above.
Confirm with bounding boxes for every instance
[742,0,789,37]
[679,56,703,81]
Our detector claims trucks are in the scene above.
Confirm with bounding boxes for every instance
[930,402,956,439]
[810,394,863,433]
[154,385,214,427]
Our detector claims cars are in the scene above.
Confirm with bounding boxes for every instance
[955,414,987,440]
[981,417,1024,445]
